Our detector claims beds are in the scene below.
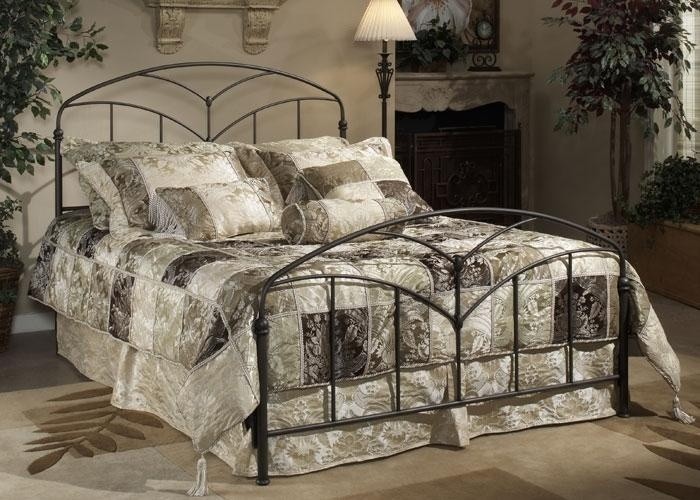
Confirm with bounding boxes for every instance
[39,57,639,492]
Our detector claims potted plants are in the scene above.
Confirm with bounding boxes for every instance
[540,1,700,260]
[1,0,111,352]
[631,151,700,309]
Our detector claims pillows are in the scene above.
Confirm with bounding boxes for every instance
[148,177,283,243]
[280,197,408,245]
[60,131,445,228]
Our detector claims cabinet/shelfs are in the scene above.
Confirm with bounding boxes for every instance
[394,123,523,227]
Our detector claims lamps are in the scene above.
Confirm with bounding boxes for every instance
[352,0,420,139]
